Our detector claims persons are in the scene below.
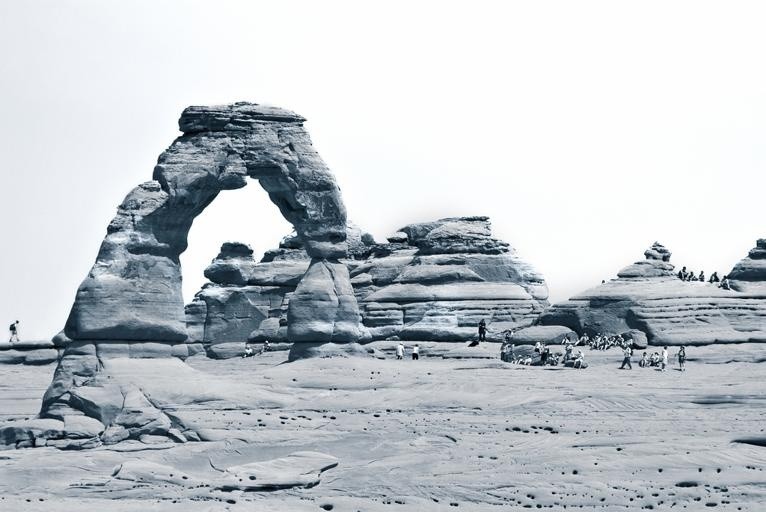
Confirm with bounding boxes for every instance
[505,329,687,371]
[412,344,419,360]
[679,266,729,289]
[9,320,20,342]
[478,319,486,342]
[396,342,405,359]
[243,340,271,357]
